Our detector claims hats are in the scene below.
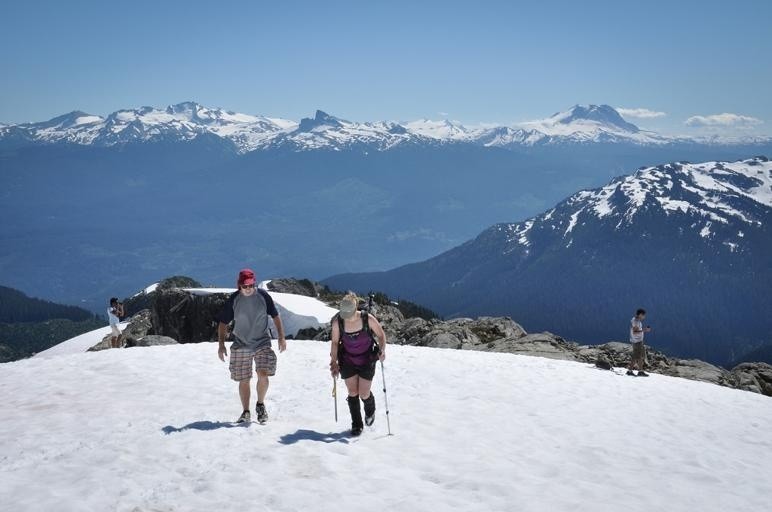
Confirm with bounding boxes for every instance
[339,297,356,319]
[239,269,256,285]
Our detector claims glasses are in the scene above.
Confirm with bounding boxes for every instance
[240,285,254,288]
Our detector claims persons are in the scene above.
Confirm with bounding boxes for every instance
[626,308,652,376]
[107,298,124,348]
[329,291,387,437]
[216,269,287,424]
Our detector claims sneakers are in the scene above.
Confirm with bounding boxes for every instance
[255,401,269,423]
[237,410,250,423]
[365,413,375,426]
[627,371,649,377]
[352,426,363,435]
